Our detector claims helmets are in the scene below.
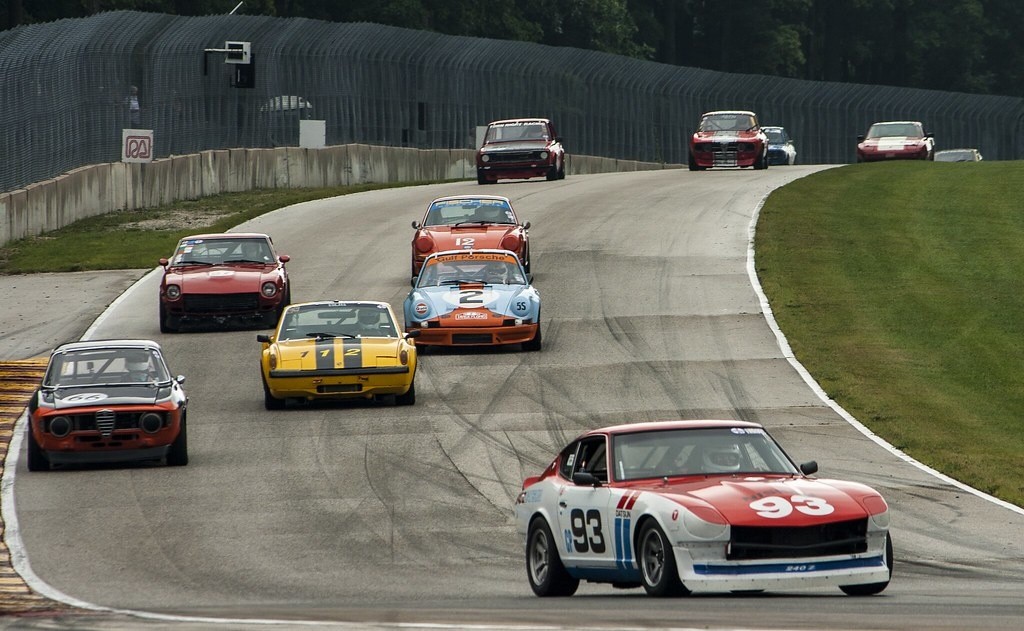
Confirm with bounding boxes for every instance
[481,206,500,218]
[243,242,260,257]
[702,442,741,472]
[486,261,508,281]
[358,309,381,330]
[125,353,150,371]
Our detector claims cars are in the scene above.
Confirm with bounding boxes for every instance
[255,298,423,410]
[411,194,533,284]
[26,338,189,473]
[688,110,768,170]
[157,233,292,334]
[476,118,566,185]
[760,126,798,165]
[933,149,981,164]
[857,121,936,162]
[402,249,542,352]
[513,419,893,599]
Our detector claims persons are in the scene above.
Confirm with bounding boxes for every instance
[484,262,516,284]
[479,206,510,223]
[701,442,742,473]
[529,126,544,139]
[121,354,159,382]
[240,242,269,261]
[731,118,751,131]
[350,309,390,338]
